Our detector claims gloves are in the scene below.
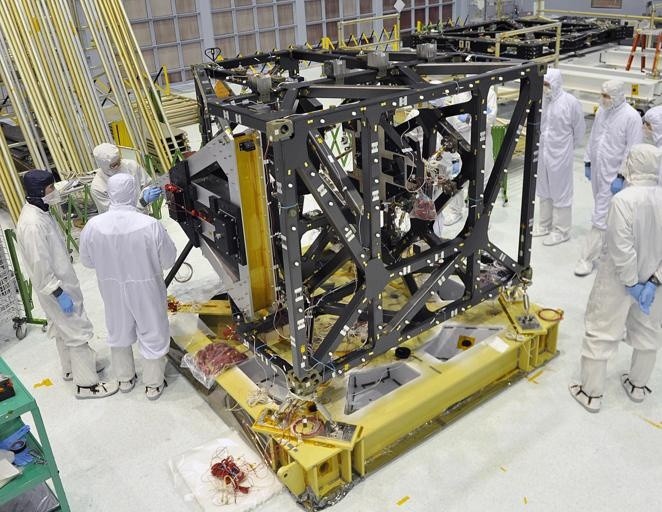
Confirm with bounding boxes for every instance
[452,161,459,174]
[610,177,624,193]
[626,282,657,313]
[143,186,162,203]
[1,424,33,466]
[584,166,591,179]
[57,292,73,313]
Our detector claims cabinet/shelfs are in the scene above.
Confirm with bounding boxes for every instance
[0,356,70,512]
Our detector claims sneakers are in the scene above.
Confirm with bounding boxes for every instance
[145,377,167,400]
[574,260,593,276]
[621,370,646,402]
[569,383,602,413]
[444,211,463,226]
[119,372,137,393]
[533,222,551,237]
[62,360,104,381]
[543,230,569,245]
[75,380,119,399]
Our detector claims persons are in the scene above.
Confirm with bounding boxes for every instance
[17,169,119,399]
[533,69,586,245]
[568,143,662,412]
[644,105,662,188]
[403,74,498,244]
[79,172,176,399]
[89,142,162,214]
[575,80,643,277]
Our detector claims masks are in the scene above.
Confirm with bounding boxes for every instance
[42,188,61,205]
[640,124,650,141]
[597,98,612,111]
[543,86,551,95]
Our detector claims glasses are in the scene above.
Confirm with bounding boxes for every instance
[111,163,120,169]
